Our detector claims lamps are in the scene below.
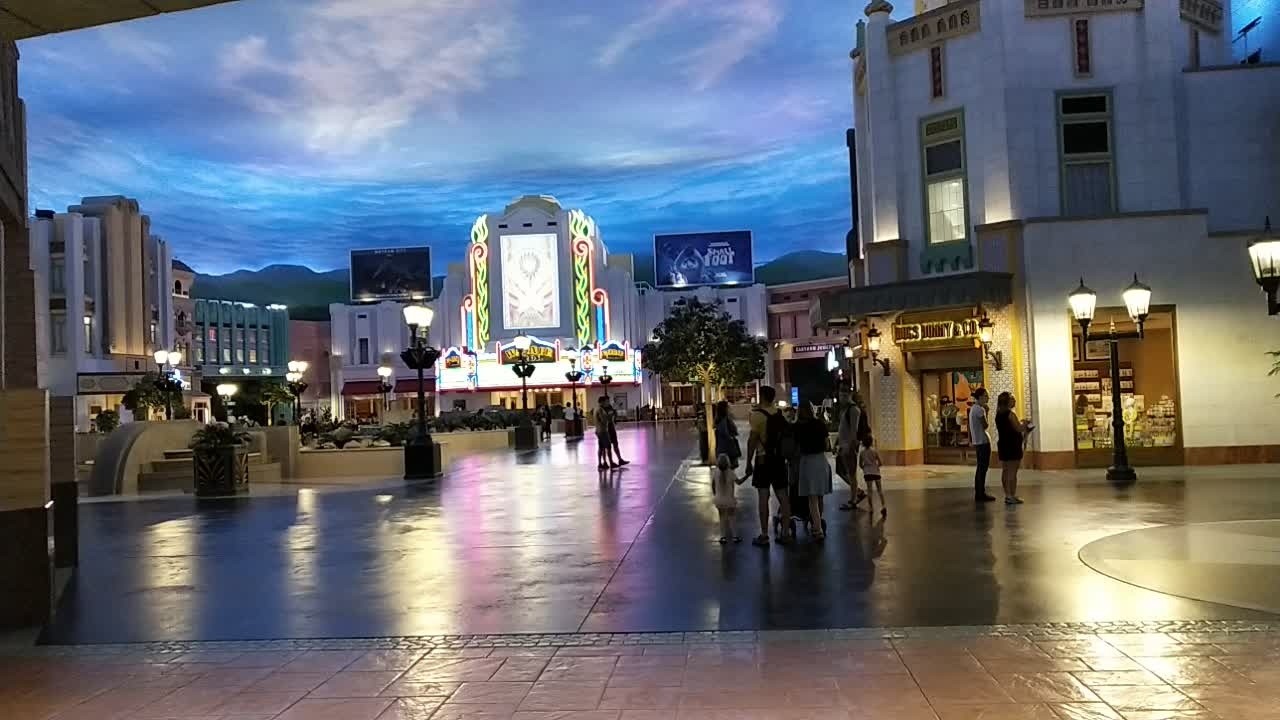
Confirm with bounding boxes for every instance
[979,311,1002,370]
[867,323,890,376]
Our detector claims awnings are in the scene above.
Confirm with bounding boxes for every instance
[393,378,436,393]
[341,381,384,395]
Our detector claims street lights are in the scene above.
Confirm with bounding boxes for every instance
[215,384,238,422]
[565,345,583,437]
[285,372,302,421]
[510,328,537,427]
[599,358,613,397]
[398,300,441,441]
[1067,269,1152,482]
[153,350,182,419]
[288,359,309,417]
[375,367,394,410]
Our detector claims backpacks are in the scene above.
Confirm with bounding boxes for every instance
[845,404,871,441]
[754,408,790,449]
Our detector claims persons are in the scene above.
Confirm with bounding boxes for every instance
[278,408,375,443]
[562,401,584,437]
[995,392,1036,504]
[591,395,630,469]
[534,404,552,441]
[969,388,996,501]
[745,385,887,546]
[710,452,752,542]
[713,401,742,470]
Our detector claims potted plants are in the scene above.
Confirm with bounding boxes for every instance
[187,420,251,496]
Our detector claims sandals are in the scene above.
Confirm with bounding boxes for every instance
[720,537,728,544]
[732,536,744,543]
[839,501,856,509]
[855,490,867,503]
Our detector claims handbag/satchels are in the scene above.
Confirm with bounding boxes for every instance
[729,436,742,457]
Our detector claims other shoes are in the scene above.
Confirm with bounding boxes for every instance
[880,507,887,519]
[811,528,825,540]
[869,508,873,513]
[775,536,793,545]
[1005,496,1024,504]
[611,463,620,468]
[619,459,629,465]
[604,463,609,468]
[598,464,602,468]
[975,495,995,501]
[752,534,768,544]
